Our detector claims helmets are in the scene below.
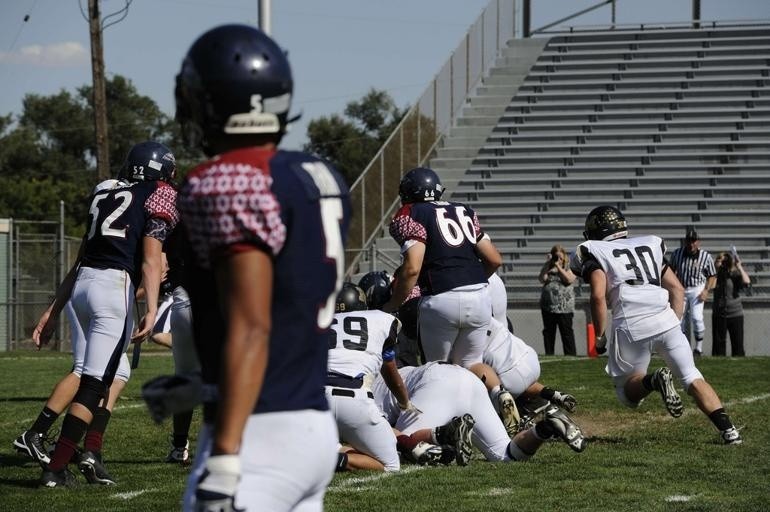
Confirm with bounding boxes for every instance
[358,269,392,312]
[173,22,294,164]
[333,281,368,312]
[580,203,632,239]
[120,142,177,180]
[397,166,446,202]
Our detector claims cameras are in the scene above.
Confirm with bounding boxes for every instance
[550,254,558,268]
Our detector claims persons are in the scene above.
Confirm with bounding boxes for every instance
[33,141,178,489]
[372,360,588,466]
[178,23,351,512]
[326,285,424,473]
[570,205,745,445]
[538,245,577,356]
[13,178,128,470]
[668,230,717,355]
[711,252,752,357]
[358,271,421,366]
[382,168,520,439]
[483,317,576,413]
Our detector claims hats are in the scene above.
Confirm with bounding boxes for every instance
[684,225,703,240]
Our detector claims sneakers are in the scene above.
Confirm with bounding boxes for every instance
[38,462,80,488]
[412,440,459,468]
[11,425,55,466]
[452,412,480,470]
[543,405,590,453]
[498,390,526,436]
[653,365,686,420]
[167,446,188,464]
[77,450,118,485]
[552,391,579,408]
[717,422,742,444]
